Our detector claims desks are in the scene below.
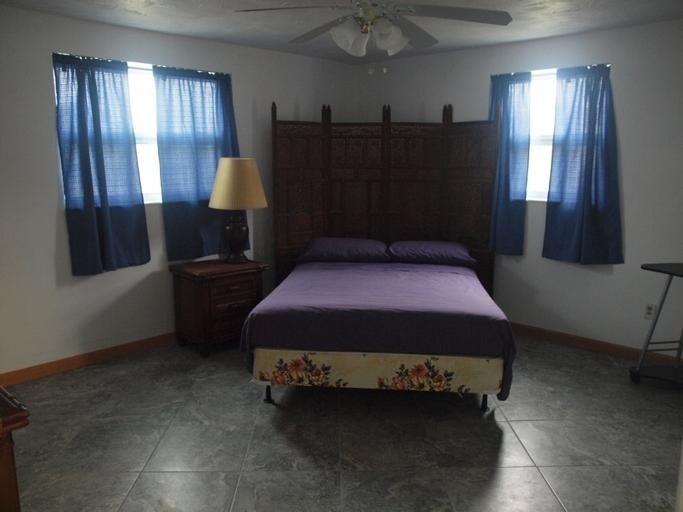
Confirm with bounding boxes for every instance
[0,385,28,511]
[628,262,682,386]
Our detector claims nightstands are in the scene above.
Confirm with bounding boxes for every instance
[169,259,271,358]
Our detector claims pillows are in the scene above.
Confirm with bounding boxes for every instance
[300,237,479,269]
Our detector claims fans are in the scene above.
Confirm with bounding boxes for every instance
[228,0,515,56]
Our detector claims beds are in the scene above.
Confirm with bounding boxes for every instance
[246,241,512,414]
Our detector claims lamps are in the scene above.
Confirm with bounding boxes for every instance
[207,155,270,266]
[326,11,411,56]
